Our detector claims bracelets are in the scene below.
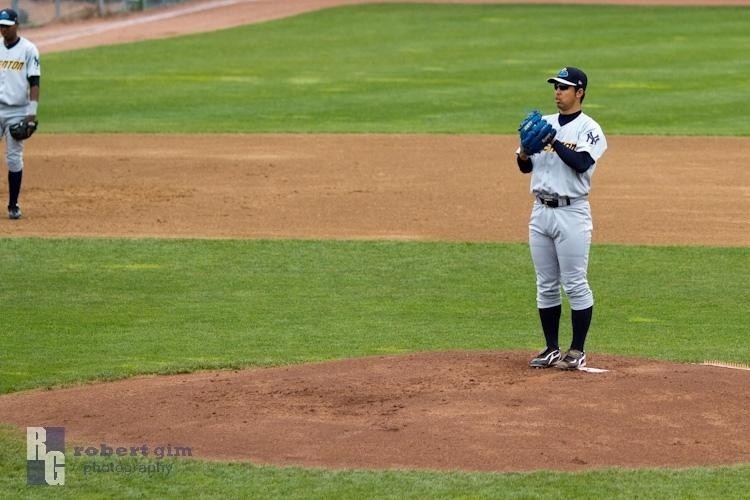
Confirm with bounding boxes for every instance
[28,101,38,115]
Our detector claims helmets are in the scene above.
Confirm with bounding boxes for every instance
[0,9,19,26]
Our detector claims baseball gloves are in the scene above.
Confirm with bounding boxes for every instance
[518,111,557,155]
[9,120,37,141]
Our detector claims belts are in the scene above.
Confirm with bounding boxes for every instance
[538,197,570,208]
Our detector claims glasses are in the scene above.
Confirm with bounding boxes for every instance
[555,83,571,90]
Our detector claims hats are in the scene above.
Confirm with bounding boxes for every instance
[546,67,587,91]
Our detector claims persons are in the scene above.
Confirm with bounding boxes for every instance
[515,67,607,370]
[0,9,41,219]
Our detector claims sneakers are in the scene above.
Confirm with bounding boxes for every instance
[529,346,562,369]
[8,203,22,218]
[556,348,587,370]
